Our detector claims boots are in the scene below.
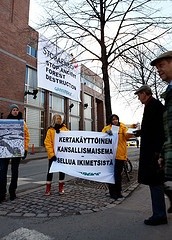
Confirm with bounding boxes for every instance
[44,181,52,196]
[59,180,65,194]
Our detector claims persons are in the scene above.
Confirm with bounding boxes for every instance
[102,115,128,200]
[134,85,172,227]
[150,51,172,194]
[0,105,30,204]
[44,114,70,195]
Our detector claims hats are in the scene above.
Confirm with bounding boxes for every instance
[9,104,19,112]
[149,51,172,66]
[134,84,152,94]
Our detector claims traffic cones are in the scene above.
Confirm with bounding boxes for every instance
[31,144,36,155]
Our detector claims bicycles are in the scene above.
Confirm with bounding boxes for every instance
[124,159,132,181]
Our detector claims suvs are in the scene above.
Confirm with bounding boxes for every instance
[126,138,139,148]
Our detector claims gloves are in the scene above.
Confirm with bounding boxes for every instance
[51,156,56,161]
[21,151,27,160]
[107,130,113,136]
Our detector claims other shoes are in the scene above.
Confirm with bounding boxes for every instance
[10,190,17,200]
[144,216,168,225]
[112,194,123,200]
[0,193,7,202]
[167,205,172,213]
